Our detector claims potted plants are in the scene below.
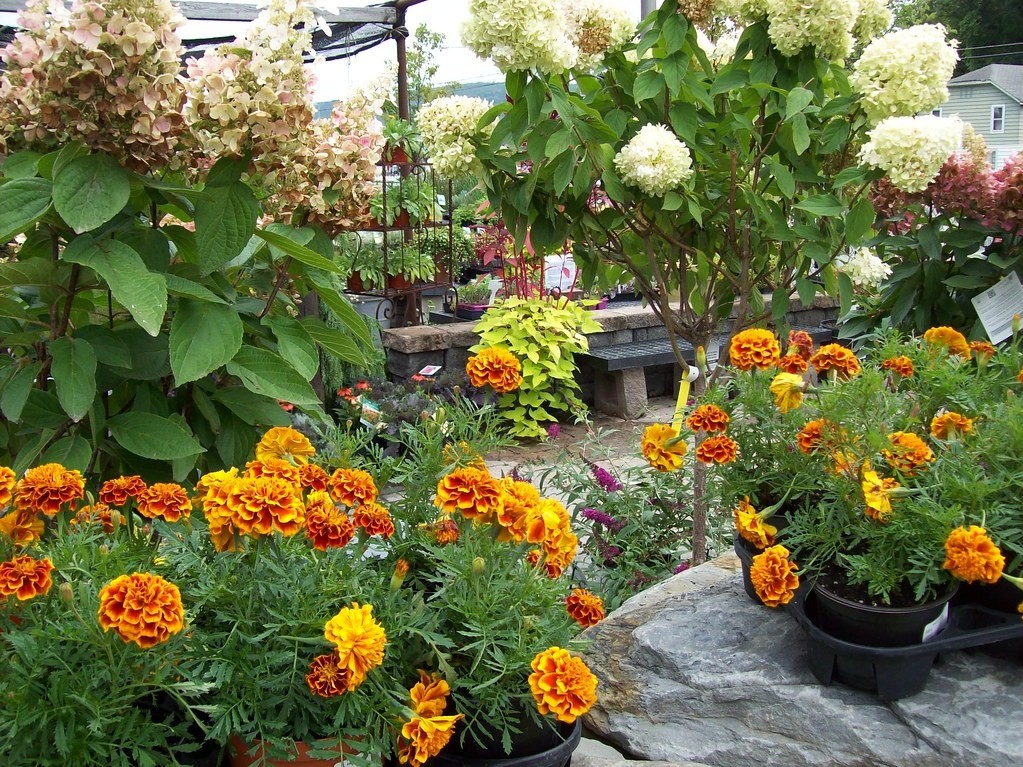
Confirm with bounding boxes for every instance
[456,278,491,321]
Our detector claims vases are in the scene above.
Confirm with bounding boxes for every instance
[390,207,410,228]
[422,205,431,225]
[601,290,643,304]
[386,672,582,767]
[803,572,964,642]
[738,465,829,519]
[460,264,476,285]
[433,251,448,283]
[391,273,410,289]
[349,269,373,292]
[732,524,761,604]
[361,207,379,229]
[216,718,384,767]
[480,267,493,276]
[590,549,673,571]
[380,144,408,164]
[105,695,224,767]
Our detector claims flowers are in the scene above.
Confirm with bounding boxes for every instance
[0,0,1023,767]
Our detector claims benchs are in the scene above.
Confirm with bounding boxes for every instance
[571,323,830,421]
